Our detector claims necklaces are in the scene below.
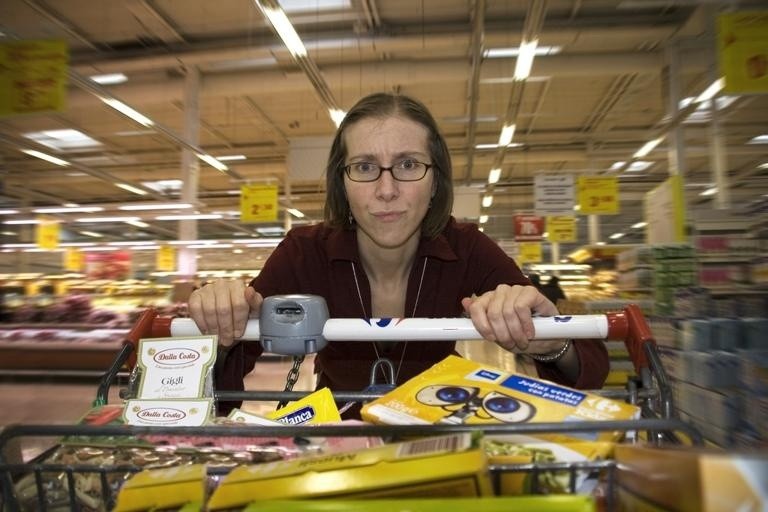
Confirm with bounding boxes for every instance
[351,255,429,385]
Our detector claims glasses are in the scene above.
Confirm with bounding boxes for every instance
[340,158,434,182]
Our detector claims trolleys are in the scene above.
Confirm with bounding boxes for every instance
[0,306,714,511]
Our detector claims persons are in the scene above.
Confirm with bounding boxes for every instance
[544,276,567,304]
[527,272,557,314]
[189,89,613,425]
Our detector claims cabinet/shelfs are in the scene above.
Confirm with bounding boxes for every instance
[558,250,768,393]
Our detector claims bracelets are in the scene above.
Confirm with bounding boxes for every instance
[519,333,572,363]
